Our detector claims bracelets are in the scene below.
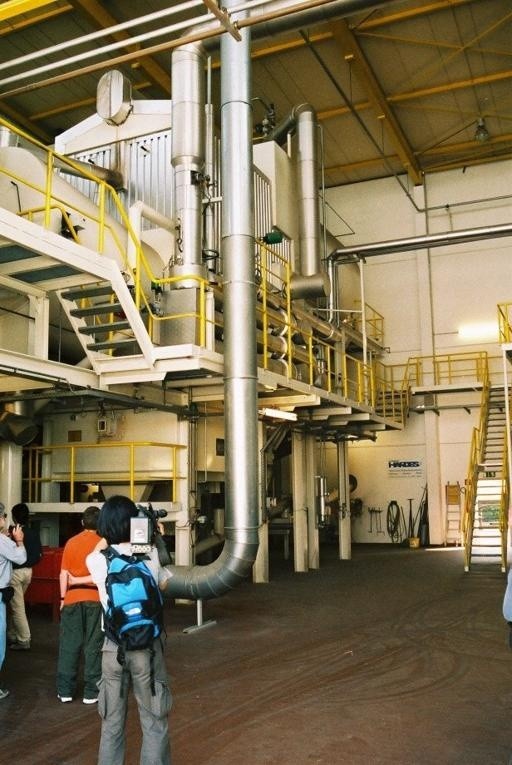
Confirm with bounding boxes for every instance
[17,540,23,543]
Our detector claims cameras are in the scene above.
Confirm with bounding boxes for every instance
[8,524,26,534]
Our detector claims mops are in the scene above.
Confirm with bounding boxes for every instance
[399,511,410,548]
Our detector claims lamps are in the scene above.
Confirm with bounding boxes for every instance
[473,118,491,141]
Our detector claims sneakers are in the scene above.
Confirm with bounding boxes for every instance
[56,693,74,702]
[10,640,31,651]
[0,690,9,699]
[83,696,98,705]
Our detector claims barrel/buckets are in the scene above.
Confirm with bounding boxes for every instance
[408,537,420,548]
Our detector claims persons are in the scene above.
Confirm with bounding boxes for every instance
[57,506,100,706]
[0,502,28,699]
[8,504,42,651]
[501,565,511,653]
[84,496,173,764]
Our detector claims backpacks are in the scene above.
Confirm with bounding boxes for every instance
[100,545,165,646]
